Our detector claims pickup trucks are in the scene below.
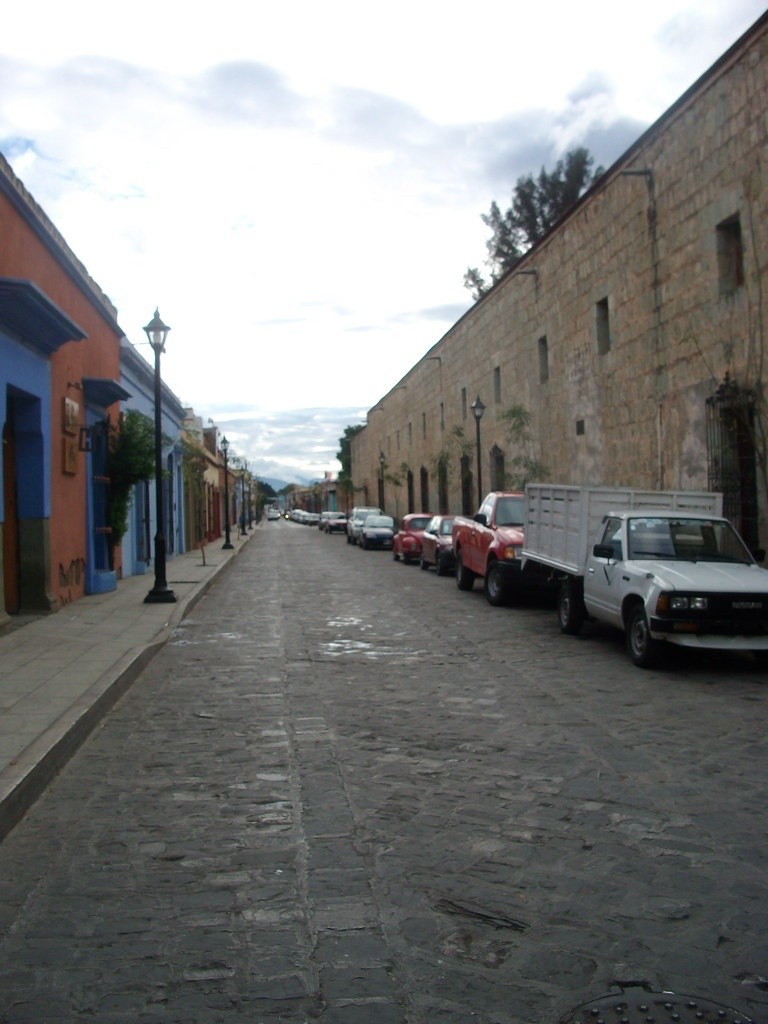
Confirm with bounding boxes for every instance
[449,490,580,607]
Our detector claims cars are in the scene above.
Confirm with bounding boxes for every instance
[284,509,320,526]
[325,513,348,534]
[359,516,396,550]
[392,513,434,563]
[319,512,333,529]
[421,515,460,575]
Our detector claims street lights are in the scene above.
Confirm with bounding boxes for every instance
[248,479,253,529]
[221,434,233,550]
[377,450,387,508]
[141,307,176,604]
[470,395,485,507]
[240,466,247,534]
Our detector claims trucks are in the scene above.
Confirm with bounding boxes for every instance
[521,479,767,667]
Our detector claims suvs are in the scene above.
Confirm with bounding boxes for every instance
[347,507,386,545]
